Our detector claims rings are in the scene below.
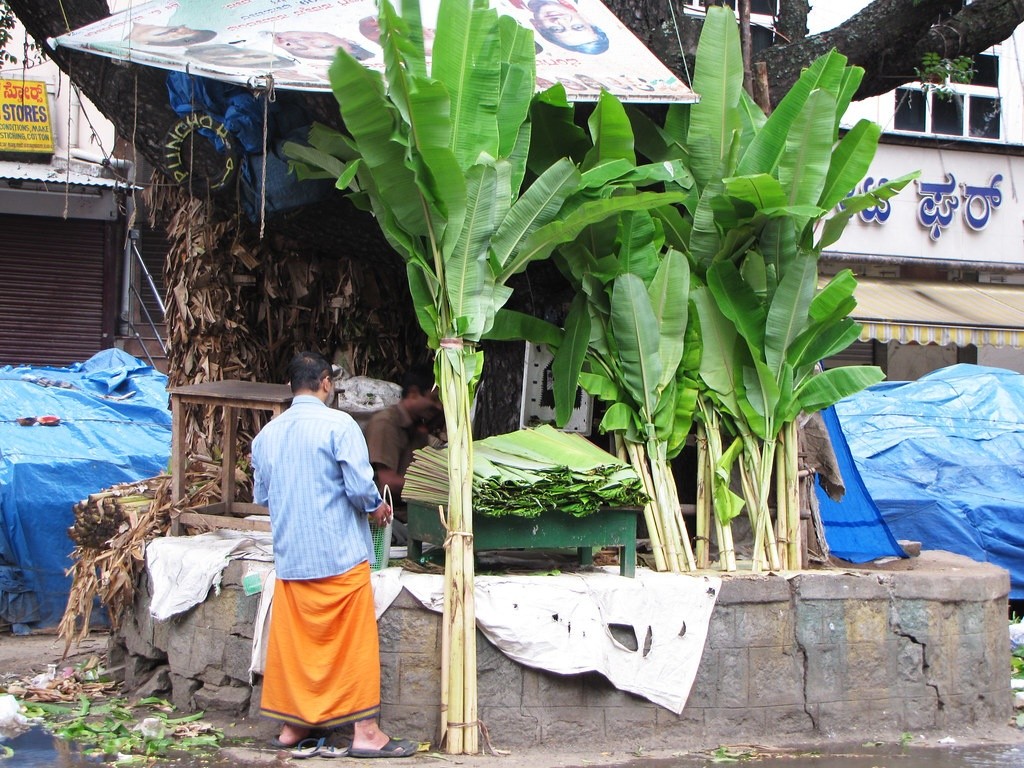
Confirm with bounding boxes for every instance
[382,517,386,522]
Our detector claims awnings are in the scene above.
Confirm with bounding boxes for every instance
[817,275,1024,350]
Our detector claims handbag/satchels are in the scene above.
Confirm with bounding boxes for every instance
[368,484,393,571]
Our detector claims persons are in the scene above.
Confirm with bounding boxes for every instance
[89,0,608,70]
[364,371,443,522]
[251,353,419,759]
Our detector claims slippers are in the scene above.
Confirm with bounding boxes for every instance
[321,736,353,758]
[291,738,337,758]
[349,735,418,758]
[271,732,320,747]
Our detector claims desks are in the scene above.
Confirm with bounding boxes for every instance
[401,496,646,580]
[167,378,347,538]
